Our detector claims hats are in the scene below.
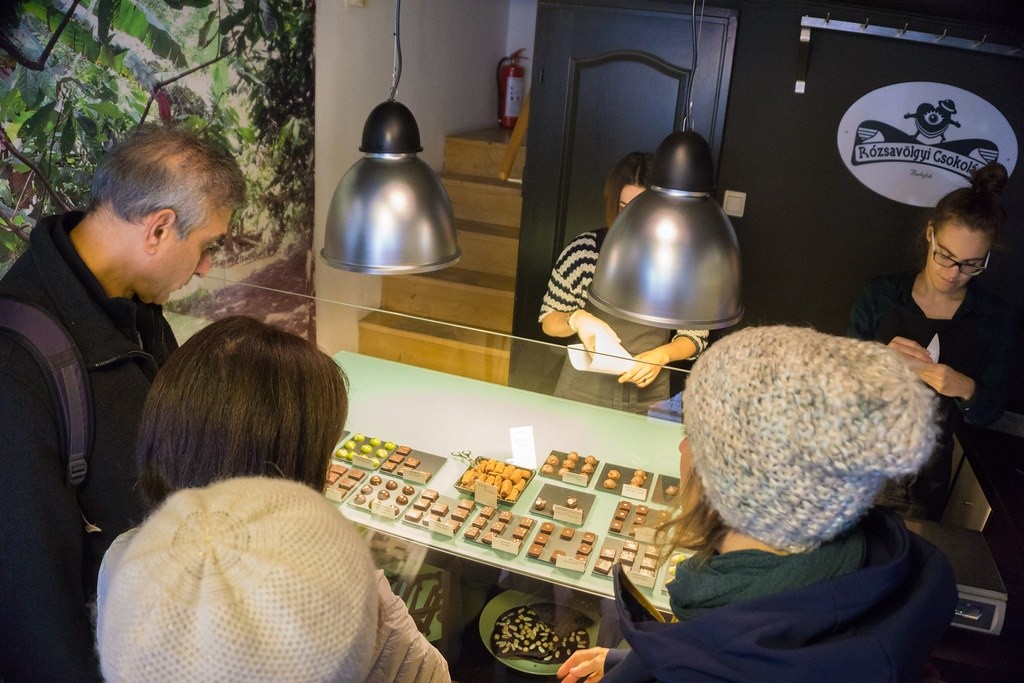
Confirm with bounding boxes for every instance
[683,325,939,553]
[95,476,380,683]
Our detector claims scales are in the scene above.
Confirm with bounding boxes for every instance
[898,516,1008,637]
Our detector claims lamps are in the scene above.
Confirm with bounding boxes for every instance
[320,0,463,275]
[586,0,745,330]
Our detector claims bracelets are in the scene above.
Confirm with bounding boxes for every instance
[567,311,576,334]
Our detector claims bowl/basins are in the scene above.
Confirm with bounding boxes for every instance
[451,456,537,508]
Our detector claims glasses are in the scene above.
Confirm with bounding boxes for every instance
[931,226,990,276]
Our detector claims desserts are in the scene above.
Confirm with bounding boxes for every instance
[491,604,594,664]
[323,433,687,581]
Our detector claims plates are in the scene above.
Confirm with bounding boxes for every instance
[480,589,632,676]
[379,561,486,642]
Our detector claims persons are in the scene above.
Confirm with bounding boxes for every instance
[98,477,377,683]
[849,164,1018,425]
[134,315,453,683]
[556,324,957,682]
[0,124,246,683]
[539,152,710,414]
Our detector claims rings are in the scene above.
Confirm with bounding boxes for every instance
[643,377,646,381]
